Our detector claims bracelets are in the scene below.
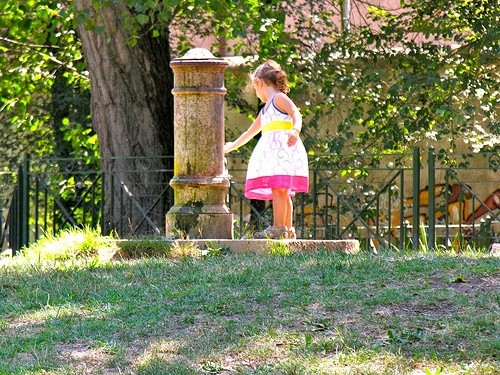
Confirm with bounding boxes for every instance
[293,128,301,134]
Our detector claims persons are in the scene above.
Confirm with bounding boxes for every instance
[224,60,309,239]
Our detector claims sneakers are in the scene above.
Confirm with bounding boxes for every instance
[288,227,296,239]
[254,225,288,239]
